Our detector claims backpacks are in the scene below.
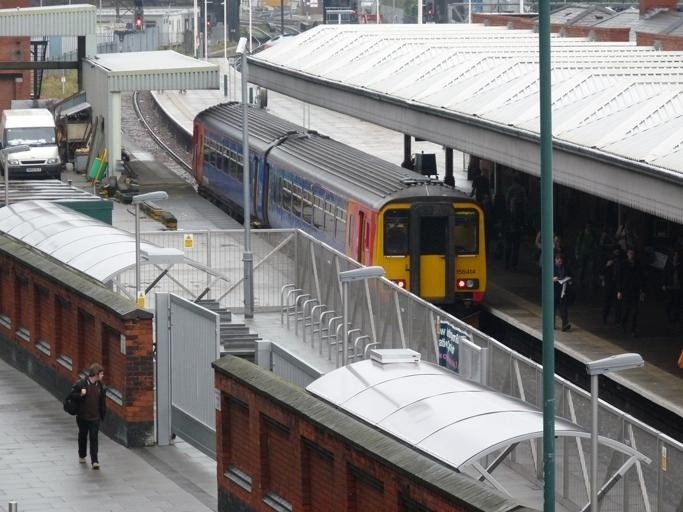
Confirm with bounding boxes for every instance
[62,383,86,415]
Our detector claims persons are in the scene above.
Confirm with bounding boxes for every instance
[660,249,682,336]
[535,212,648,338]
[63,362,106,469]
[470,168,523,270]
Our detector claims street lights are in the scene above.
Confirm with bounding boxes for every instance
[130,190,175,306]
[584,351,647,512]
[0,142,31,207]
[232,36,256,317]
[338,265,385,369]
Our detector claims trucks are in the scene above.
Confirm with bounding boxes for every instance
[0,107,66,182]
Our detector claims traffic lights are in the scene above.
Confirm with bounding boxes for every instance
[134,9,142,28]
[206,16,211,31]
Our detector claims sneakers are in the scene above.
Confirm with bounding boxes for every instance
[78,456,86,462]
[562,323,570,331]
[92,462,99,469]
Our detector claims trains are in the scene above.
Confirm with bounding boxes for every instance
[186,96,491,325]
